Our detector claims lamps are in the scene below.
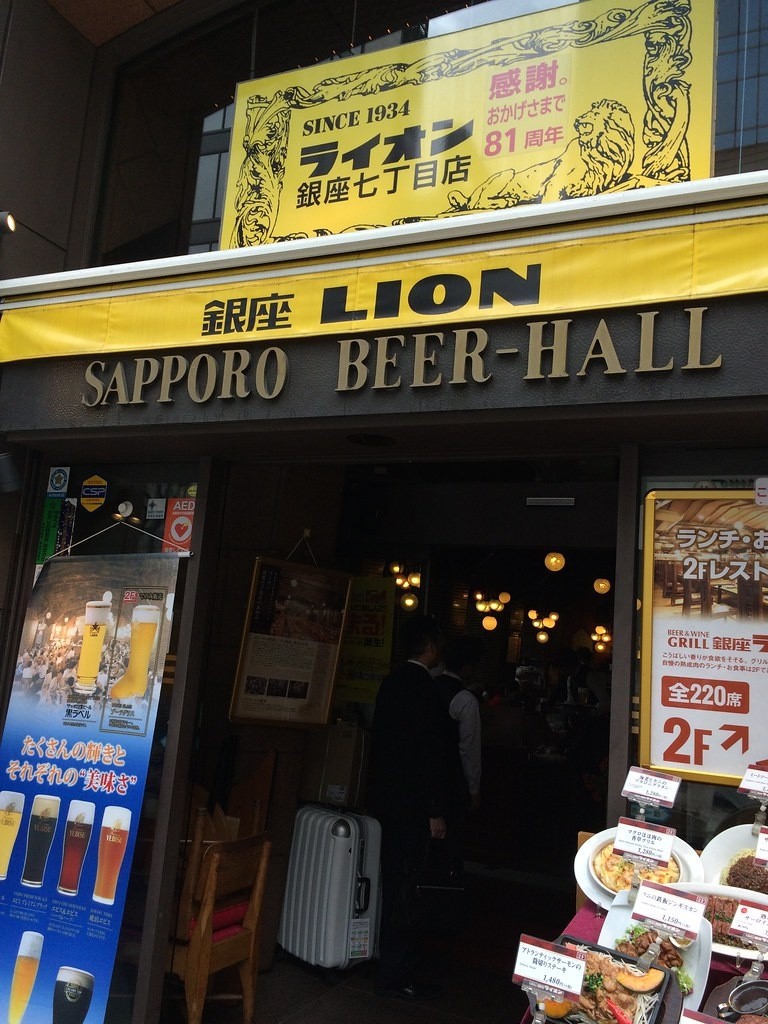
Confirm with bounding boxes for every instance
[389,562,421,612]
[590,625,613,657]
[0,211,16,234]
[543,546,566,572]
[636,598,642,610]
[593,576,610,596]
[528,608,561,645]
[473,589,512,632]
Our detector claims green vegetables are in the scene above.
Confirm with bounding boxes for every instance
[616,924,647,946]
[668,965,693,995]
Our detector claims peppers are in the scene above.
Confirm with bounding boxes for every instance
[606,999,633,1024]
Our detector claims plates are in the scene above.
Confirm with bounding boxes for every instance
[698,823,768,885]
[527,933,670,1024]
[658,883,767,961]
[574,827,705,911]
[589,837,682,895]
[597,890,713,1011]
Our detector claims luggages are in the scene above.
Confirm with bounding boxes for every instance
[276,805,386,978]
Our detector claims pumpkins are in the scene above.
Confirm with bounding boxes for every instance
[535,999,576,1018]
[616,968,665,993]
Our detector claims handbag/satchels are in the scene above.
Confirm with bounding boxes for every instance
[394,830,477,940]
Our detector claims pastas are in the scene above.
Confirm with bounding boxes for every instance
[721,849,756,885]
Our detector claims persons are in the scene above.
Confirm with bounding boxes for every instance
[558,651,610,717]
[363,615,479,1001]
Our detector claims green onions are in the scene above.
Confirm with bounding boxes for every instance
[584,968,604,994]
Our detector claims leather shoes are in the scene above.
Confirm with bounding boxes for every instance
[376,973,443,1001]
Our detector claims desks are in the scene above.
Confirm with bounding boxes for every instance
[507,900,768,1024]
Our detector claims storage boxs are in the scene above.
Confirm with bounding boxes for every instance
[298,722,368,808]
[531,935,672,1024]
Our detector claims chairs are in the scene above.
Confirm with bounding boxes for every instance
[177,799,263,940]
[169,830,272,1024]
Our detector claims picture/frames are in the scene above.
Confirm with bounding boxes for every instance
[227,553,353,729]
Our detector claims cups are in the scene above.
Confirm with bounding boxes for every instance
[92,806,131,906]
[52,968,94,1024]
[57,800,95,897]
[0,791,24,881]
[577,687,587,705]
[20,795,60,888]
[74,600,113,694]
[8,931,44,1024]
[110,605,160,698]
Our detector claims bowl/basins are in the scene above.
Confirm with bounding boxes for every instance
[728,980,768,1014]
